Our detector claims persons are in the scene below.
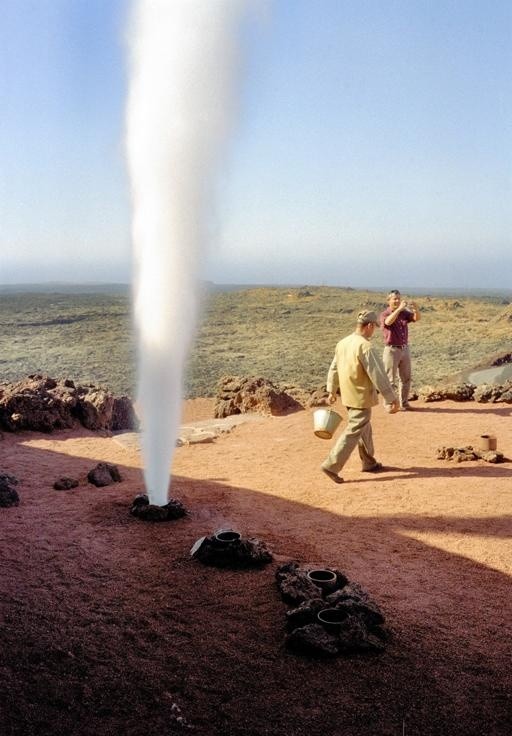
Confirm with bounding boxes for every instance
[320,310,400,484]
[379,289,421,413]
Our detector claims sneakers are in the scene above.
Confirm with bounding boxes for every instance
[321,465,344,484]
[362,462,383,472]
[401,402,414,411]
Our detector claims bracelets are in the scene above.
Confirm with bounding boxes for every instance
[413,308,419,313]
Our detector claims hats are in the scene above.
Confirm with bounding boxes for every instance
[356,310,380,328]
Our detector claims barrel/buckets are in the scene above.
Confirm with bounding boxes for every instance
[314,409,342,440]
